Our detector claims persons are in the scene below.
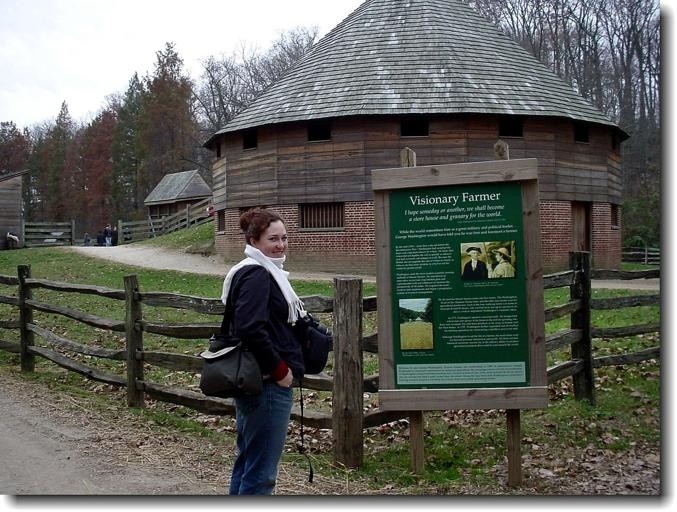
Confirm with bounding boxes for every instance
[221,206,307,495]
[492,247,516,278]
[461,247,488,279]
[84,224,132,246]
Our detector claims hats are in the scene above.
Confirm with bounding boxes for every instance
[492,248,511,258]
[466,247,482,255]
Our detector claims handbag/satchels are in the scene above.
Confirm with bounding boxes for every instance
[198,335,262,397]
[302,314,333,373]
